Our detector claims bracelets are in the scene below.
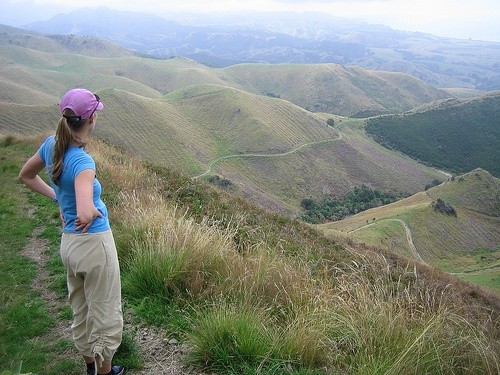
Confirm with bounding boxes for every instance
[53,198,58,202]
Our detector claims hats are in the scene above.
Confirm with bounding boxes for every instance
[58,88,105,122]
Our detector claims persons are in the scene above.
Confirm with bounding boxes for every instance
[18,88,124,375]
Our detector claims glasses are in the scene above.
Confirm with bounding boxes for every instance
[87,93,100,119]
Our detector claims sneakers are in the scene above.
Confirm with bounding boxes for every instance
[108,365,124,375]
